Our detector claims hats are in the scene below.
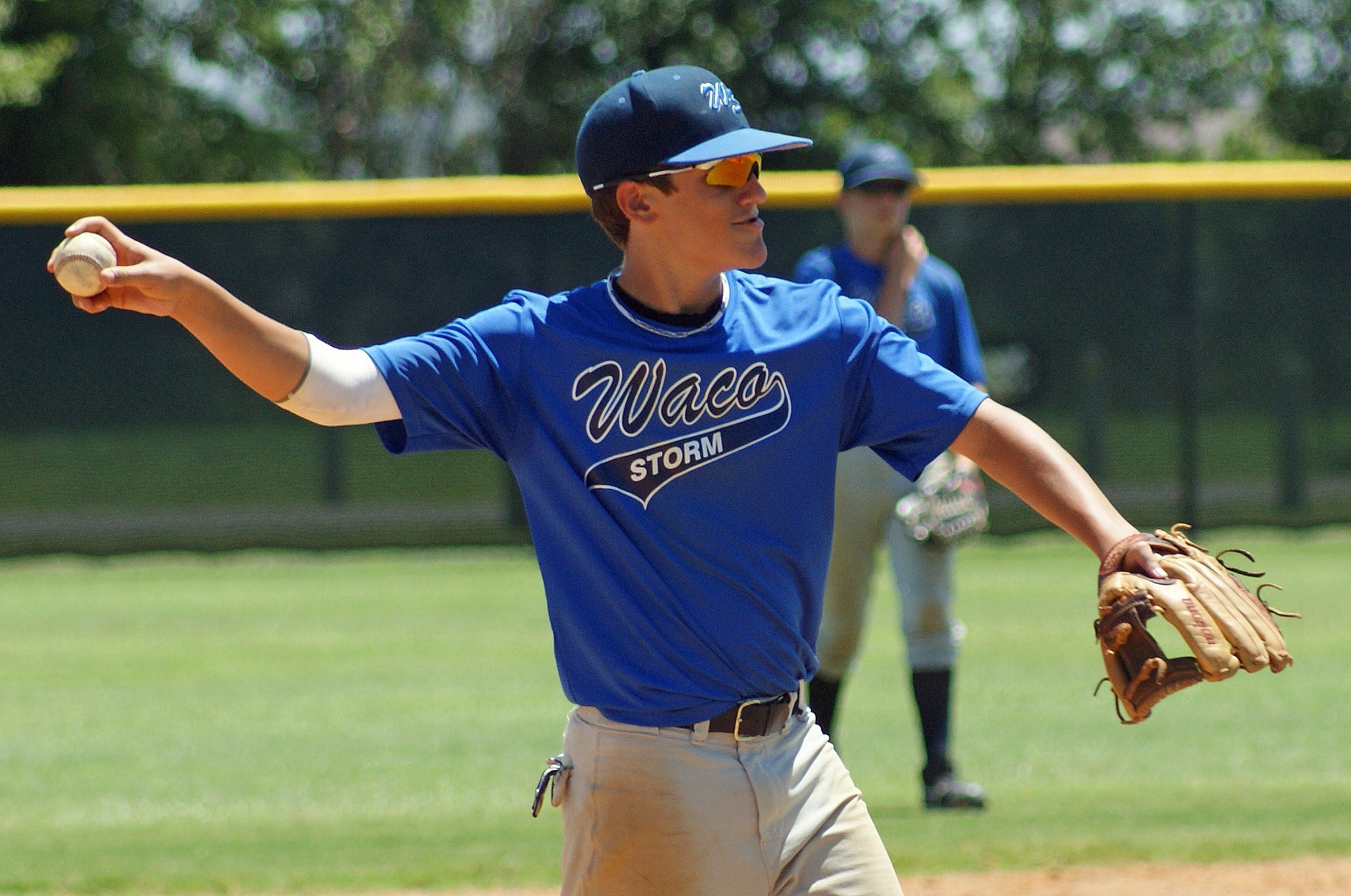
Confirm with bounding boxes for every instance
[841,138,922,197]
[574,66,813,192]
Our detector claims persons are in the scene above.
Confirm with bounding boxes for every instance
[791,140,990,809]
[44,65,1293,896]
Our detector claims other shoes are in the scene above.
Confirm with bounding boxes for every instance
[927,778,982,811]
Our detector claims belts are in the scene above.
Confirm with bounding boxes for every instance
[679,684,806,742]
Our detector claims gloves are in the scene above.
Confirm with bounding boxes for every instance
[532,757,571,817]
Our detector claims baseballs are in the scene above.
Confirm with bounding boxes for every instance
[53,232,119,296]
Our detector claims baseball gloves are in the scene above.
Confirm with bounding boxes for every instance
[1096,533,1292,721]
[893,467,993,550]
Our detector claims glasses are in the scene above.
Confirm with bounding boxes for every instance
[587,150,762,195]
